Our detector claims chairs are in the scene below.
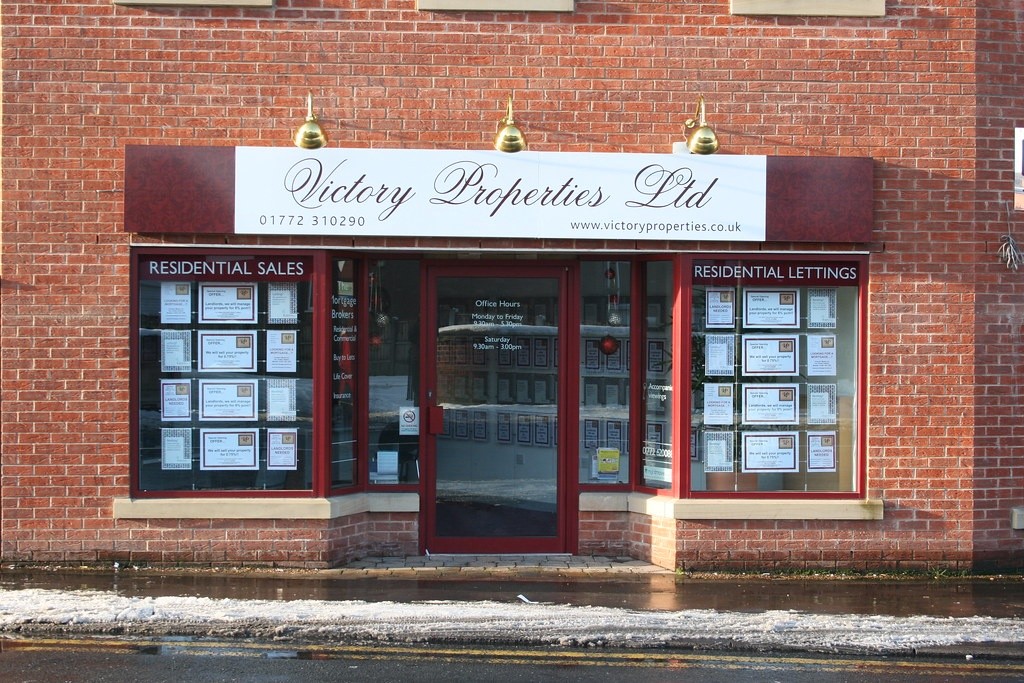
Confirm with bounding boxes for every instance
[380,422,420,482]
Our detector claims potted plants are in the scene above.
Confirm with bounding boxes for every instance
[662,311,757,490]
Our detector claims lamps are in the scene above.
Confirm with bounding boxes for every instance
[496,94,527,152]
[294,92,326,149]
[688,97,717,154]
